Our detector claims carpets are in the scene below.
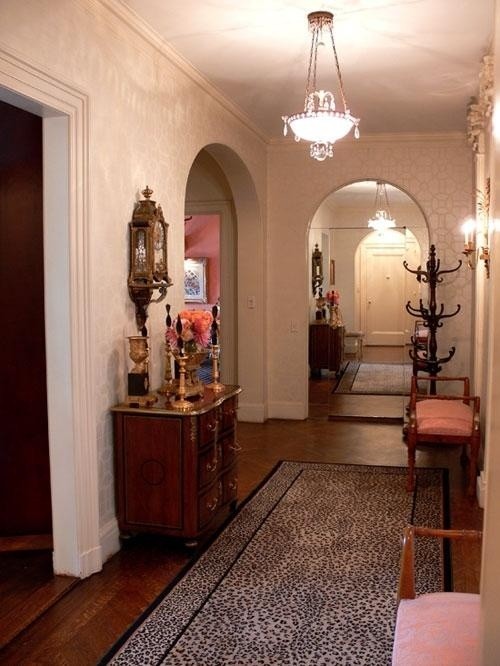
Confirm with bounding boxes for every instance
[96,457,454,666]
[332,359,427,398]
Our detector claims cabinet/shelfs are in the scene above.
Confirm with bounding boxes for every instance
[108,394,240,547]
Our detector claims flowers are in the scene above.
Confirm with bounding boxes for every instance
[166,308,212,349]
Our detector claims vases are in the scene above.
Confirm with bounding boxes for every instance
[173,350,211,387]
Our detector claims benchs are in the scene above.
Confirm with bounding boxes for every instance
[405,373,481,496]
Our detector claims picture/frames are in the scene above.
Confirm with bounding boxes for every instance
[185,256,209,305]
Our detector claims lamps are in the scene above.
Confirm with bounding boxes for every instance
[282,9,362,162]
